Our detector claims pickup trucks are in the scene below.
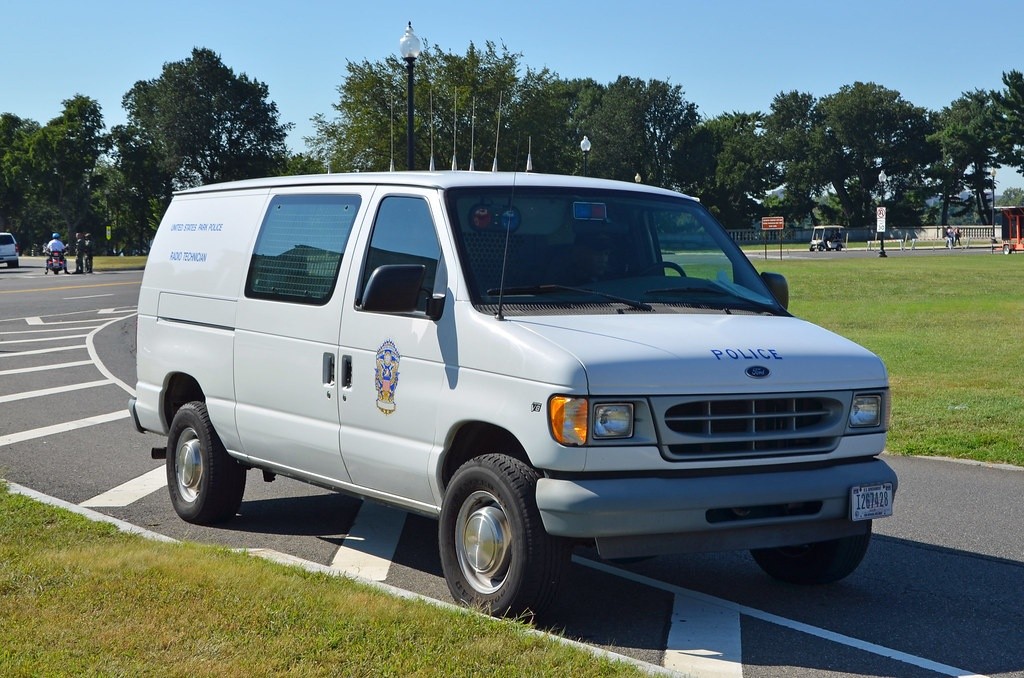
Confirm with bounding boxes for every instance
[130,169,896,623]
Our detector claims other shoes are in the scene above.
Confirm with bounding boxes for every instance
[45,271,47,274]
[87,271,92,273]
[65,271,70,274]
[72,271,82,274]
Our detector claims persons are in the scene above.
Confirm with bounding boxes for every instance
[570,232,616,282]
[72,233,85,274]
[44,233,70,274]
[418,263,493,312]
[835,229,841,239]
[829,231,835,240]
[946,226,962,247]
[84,233,95,273]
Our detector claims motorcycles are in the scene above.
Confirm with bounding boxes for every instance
[43,243,69,274]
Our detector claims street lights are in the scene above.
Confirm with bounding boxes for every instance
[878,170,887,257]
[580,135,591,176]
[990,167,998,244]
[399,20,421,172]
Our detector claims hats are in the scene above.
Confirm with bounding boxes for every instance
[85,233,91,238]
[574,230,617,252]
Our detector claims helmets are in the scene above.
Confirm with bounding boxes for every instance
[52,233,60,238]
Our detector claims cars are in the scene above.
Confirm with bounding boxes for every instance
[0,232,20,268]
